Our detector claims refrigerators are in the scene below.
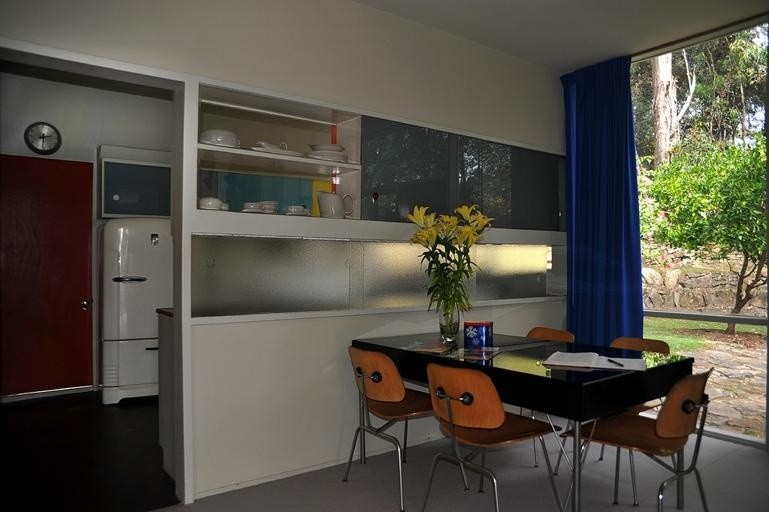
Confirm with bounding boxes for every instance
[101,217,172,404]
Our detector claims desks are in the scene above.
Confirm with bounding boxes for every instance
[352,315,693,510]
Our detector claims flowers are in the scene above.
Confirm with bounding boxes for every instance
[409,198,483,312]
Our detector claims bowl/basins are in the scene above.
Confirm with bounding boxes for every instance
[309,140,346,151]
[200,128,238,147]
[306,150,350,162]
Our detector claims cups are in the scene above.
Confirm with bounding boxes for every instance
[262,200,279,210]
[222,202,230,210]
[200,196,221,208]
[288,205,304,213]
[243,202,260,209]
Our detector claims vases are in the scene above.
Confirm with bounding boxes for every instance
[436,298,462,346]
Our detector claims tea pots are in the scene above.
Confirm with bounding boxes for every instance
[315,189,354,218]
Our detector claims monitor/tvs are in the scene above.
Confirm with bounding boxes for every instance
[101,157,172,218]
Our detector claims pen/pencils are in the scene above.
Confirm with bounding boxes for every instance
[608,359,624,367]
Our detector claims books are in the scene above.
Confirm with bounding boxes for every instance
[541,350,648,373]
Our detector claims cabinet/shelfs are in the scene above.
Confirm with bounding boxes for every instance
[159,83,362,511]
[358,110,571,461]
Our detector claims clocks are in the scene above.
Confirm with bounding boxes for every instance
[24,120,62,155]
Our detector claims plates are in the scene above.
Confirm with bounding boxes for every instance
[242,208,265,213]
[198,140,236,150]
[264,209,276,214]
[287,211,307,215]
[308,155,356,166]
[249,146,304,158]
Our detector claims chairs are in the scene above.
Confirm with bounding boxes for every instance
[423,364,564,510]
[554,336,670,508]
[514,326,576,470]
[342,342,434,512]
[560,368,716,510]
